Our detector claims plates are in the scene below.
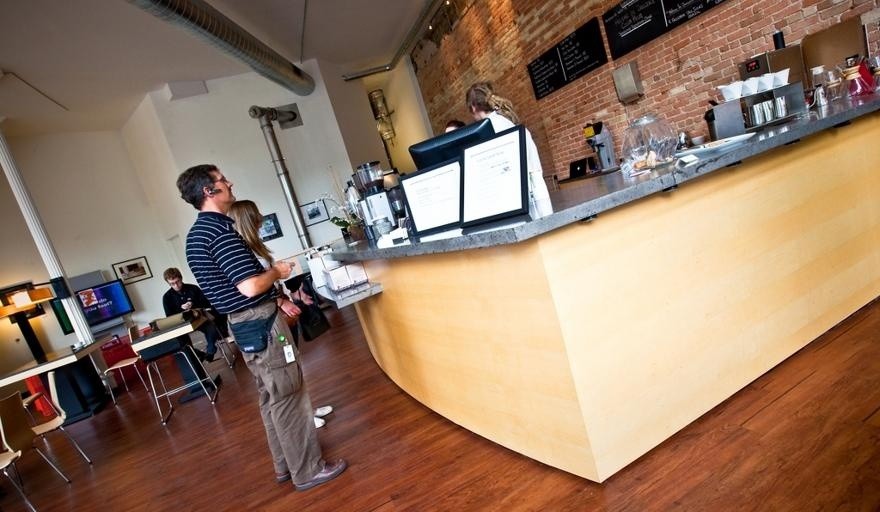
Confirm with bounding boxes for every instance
[73,342,87,350]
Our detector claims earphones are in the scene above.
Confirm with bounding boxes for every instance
[209,188,222,194]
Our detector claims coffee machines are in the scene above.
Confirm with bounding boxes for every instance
[350,160,405,229]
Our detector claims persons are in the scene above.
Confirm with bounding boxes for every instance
[444,120,466,134]
[176,164,348,491]
[228,199,335,428]
[466,81,550,203]
[161,266,219,362]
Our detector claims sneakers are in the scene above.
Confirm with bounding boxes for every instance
[206,352,214,362]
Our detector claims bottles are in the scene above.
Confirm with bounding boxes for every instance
[810,65,876,108]
[620,116,679,173]
[371,217,394,241]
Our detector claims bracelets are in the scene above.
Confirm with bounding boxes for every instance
[278,298,284,308]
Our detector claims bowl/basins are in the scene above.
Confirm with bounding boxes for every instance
[716,67,791,103]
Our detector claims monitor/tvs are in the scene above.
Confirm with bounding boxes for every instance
[48,279,135,335]
[409,118,495,170]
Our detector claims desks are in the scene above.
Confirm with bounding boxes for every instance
[131,317,218,425]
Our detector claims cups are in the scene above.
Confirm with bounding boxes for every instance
[74,341,83,348]
[746,95,789,127]
[691,135,705,146]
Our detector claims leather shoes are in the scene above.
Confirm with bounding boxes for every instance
[277,458,347,491]
[312,405,333,429]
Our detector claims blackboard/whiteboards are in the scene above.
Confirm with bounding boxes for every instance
[601,0,727,62]
[525,15,607,101]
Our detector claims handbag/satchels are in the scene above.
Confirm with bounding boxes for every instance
[295,299,331,341]
[230,317,269,353]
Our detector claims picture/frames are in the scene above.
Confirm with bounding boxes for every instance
[300,198,331,227]
[112,256,153,286]
[398,119,531,235]
[258,211,284,242]
